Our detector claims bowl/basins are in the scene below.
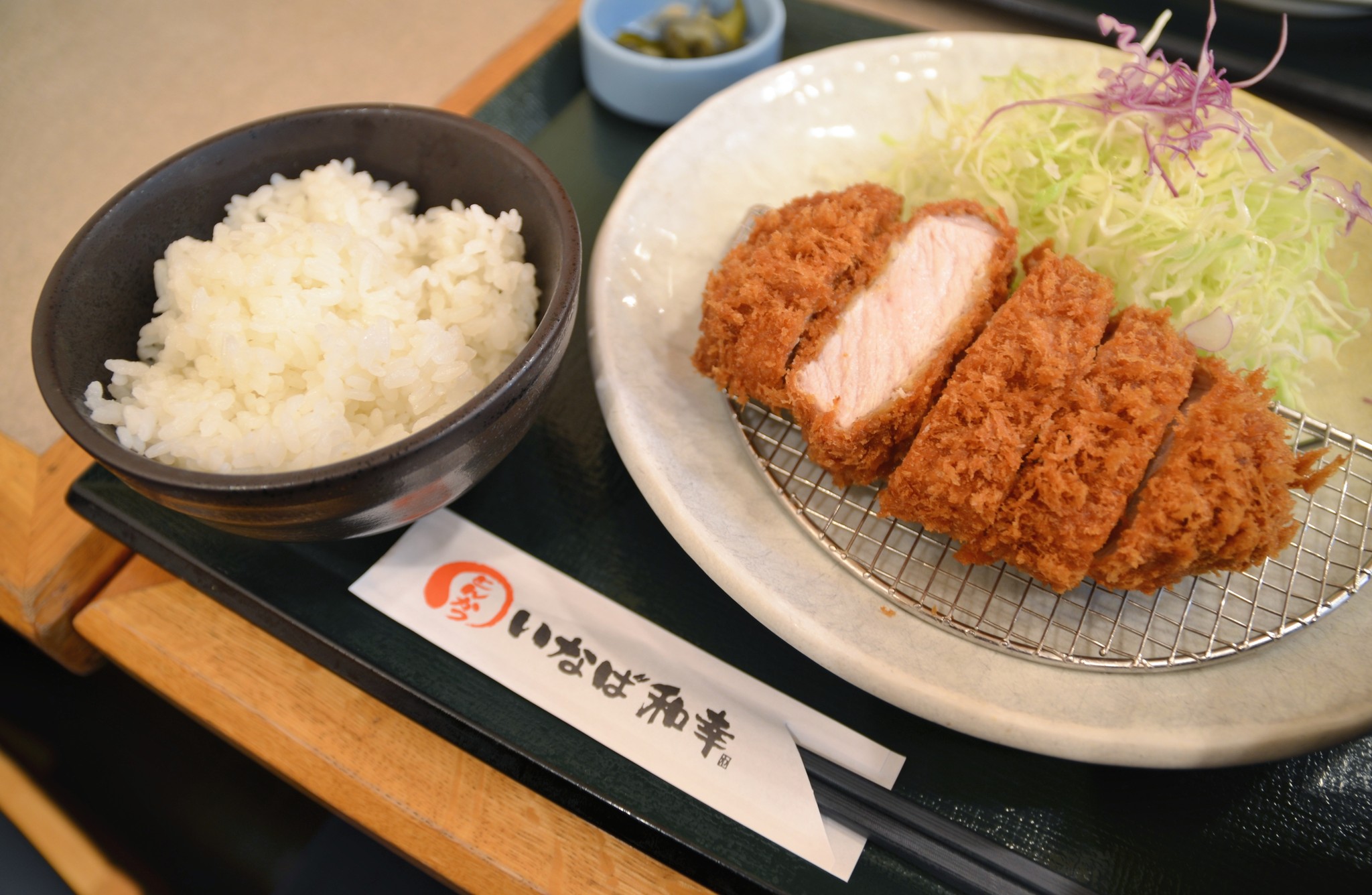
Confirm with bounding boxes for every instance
[29,104,586,543]
[580,0,789,125]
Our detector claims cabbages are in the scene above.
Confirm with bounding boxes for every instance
[858,1,1372,417]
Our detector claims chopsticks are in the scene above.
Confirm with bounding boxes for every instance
[792,740,1106,895]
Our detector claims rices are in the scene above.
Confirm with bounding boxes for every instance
[84,158,542,475]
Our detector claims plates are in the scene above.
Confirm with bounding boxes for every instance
[586,32,1372,772]
